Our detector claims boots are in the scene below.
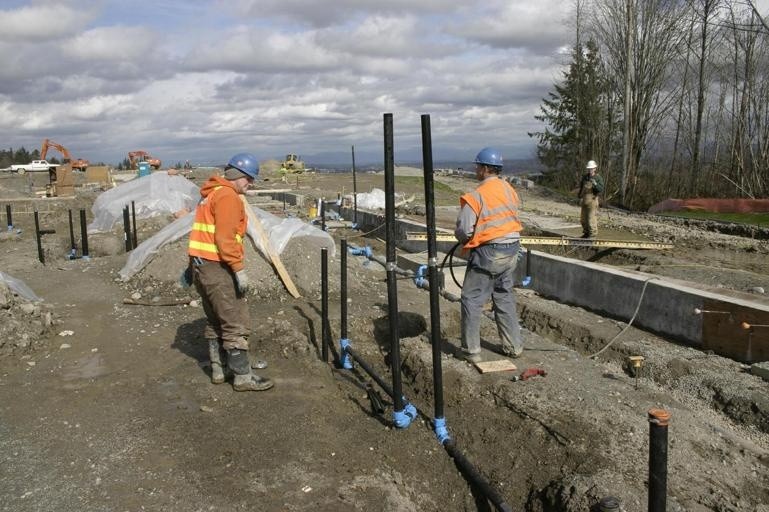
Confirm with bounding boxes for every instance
[208,338,274,392]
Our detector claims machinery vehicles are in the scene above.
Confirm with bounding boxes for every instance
[128,150,161,169]
[40,138,89,172]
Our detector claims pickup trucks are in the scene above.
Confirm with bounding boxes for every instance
[10,159,52,174]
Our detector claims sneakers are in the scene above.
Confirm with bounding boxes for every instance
[455,347,482,363]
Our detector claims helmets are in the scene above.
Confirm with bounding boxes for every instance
[229,152,259,179]
[586,160,598,169]
[472,146,504,166]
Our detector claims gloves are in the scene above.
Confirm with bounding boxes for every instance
[234,270,250,294]
[178,266,193,291]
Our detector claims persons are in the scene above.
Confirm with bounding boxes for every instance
[577,160,604,239]
[187,153,274,391]
[454,146,523,362]
